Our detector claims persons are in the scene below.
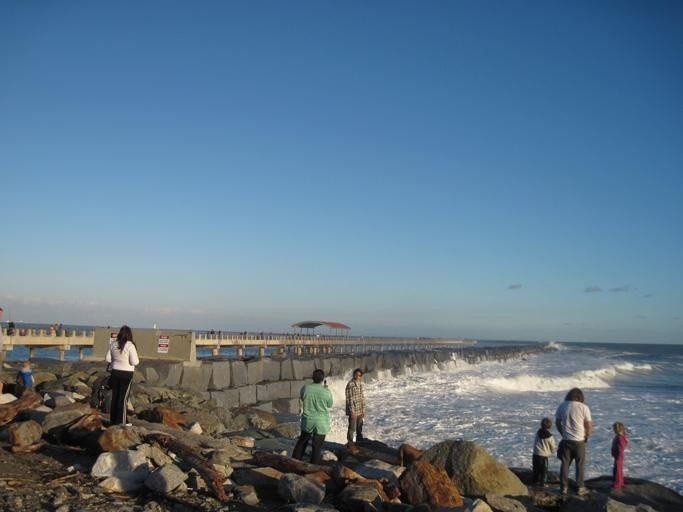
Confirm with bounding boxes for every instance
[291,368,333,465]
[609,421,628,488]
[104,324,138,427]
[555,387,592,495]
[345,367,366,445]
[0,308,4,394]
[16,361,35,387]
[90,373,113,414]
[531,417,556,487]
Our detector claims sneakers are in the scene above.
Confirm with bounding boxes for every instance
[542,482,552,488]
[125,422,132,427]
[559,488,567,497]
[577,487,589,496]
[347,445,359,454]
